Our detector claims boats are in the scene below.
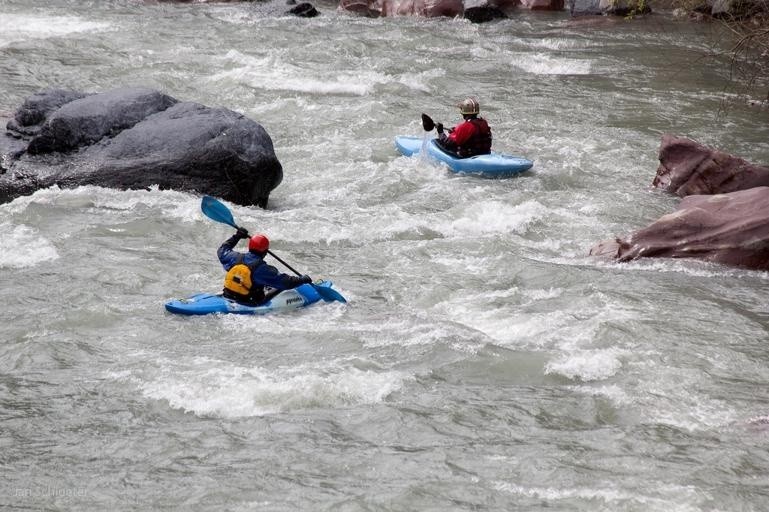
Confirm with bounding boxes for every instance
[395,132,534,179]
[162,277,335,317]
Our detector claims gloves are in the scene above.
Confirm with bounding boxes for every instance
[302,274,312,284]
[236,227,248,238]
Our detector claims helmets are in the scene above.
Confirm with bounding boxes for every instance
[460,98,479,115]
[249,234,269,254]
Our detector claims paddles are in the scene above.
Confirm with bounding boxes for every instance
[422,114,450,131]
[201,195,347,303]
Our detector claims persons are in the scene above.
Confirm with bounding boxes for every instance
[437,98,491,157]
[217,226,312,307]
